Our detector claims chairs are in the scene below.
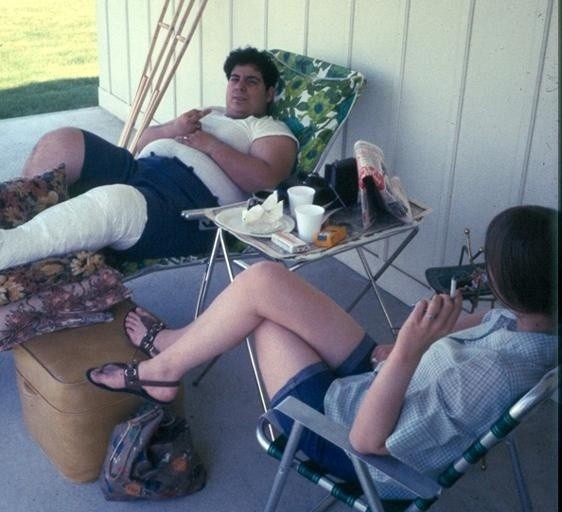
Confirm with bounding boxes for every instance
[103,48,369,325]
[253,365,558,512]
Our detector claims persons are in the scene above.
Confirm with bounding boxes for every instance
[1,44,301,268]
[86,202,561,506]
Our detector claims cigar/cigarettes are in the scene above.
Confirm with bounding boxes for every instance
[449,275,457,303]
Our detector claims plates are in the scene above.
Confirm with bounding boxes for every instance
[214,206,296,239]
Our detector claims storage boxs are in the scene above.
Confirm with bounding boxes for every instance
[13,299,186,485]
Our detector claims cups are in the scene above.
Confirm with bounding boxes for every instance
[294,205,325,243]
[287,185,316,219]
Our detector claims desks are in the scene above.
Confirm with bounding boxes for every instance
[193,189,425,444]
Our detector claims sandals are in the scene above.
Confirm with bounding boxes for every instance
[122,305,170,360]
[87,358,183,405]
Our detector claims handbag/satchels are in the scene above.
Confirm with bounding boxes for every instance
[96,399,209,502]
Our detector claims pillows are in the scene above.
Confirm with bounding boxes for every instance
[0,161,134,352]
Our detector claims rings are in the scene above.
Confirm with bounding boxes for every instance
[370,356,378,363]
[183,135,189,140]
[423,312,436,320]
